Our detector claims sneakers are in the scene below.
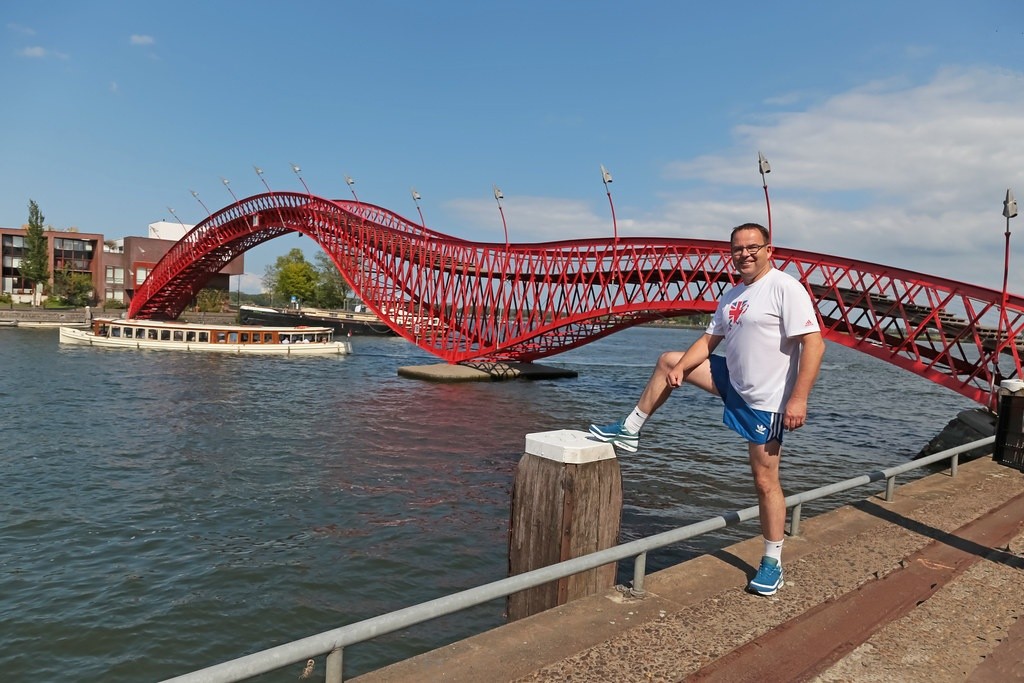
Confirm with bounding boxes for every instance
[746,556,784,595]
[589,416,639,452]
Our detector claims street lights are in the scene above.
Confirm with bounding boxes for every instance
[238,274,252,308]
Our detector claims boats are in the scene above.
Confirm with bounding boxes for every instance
[238,306,453,335]
[58,316,353,356]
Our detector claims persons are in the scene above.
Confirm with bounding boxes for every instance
[282,336,290,343]
[191,333,195,340]
[590,223,825,596]
[218,336,225,342]
[296,335,327,343]
[149,330,157,339]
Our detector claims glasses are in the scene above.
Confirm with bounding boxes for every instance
[731,245,764,255]
